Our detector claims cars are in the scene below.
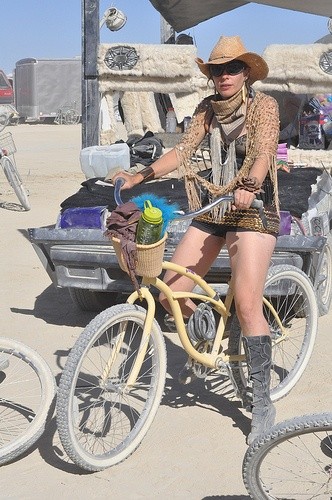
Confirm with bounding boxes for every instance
[28,144,332,319]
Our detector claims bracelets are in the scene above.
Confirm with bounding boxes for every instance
[137,167,155,183]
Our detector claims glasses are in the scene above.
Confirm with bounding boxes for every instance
[209,61,247,77]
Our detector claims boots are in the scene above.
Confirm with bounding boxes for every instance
[178,318,223,384]
[241,335,276,445]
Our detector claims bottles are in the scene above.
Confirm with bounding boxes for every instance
[134,199,162,245]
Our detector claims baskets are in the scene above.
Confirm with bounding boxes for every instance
[0,132,17,156]
[111,229,168,278]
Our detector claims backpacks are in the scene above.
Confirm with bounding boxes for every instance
[130,131,165,159]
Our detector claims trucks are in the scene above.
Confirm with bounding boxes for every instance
[0,58,82,120]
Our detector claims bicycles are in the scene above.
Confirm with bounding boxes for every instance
[241,411,332,500]
[0,113,33,212]
[56,177,318,472]
[52,100,80,126]
[0,333,58,468]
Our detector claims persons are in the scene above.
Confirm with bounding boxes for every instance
[112,35,280,444]
[176,32,193,44]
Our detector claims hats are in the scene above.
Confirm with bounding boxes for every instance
[194,35,269,80]
